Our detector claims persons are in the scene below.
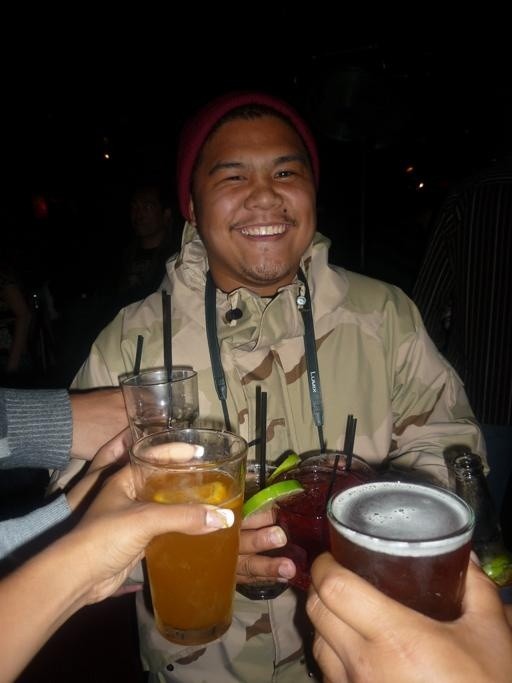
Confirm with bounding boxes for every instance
[48,93,491,683]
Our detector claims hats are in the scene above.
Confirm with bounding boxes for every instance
[178,93,321,220]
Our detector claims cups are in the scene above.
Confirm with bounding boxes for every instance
[325,476,476,625]
[269,446,376,607]
[114,368,248,648]
[238,462,292,603]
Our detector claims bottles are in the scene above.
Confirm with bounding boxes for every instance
[449,453,511,607]
